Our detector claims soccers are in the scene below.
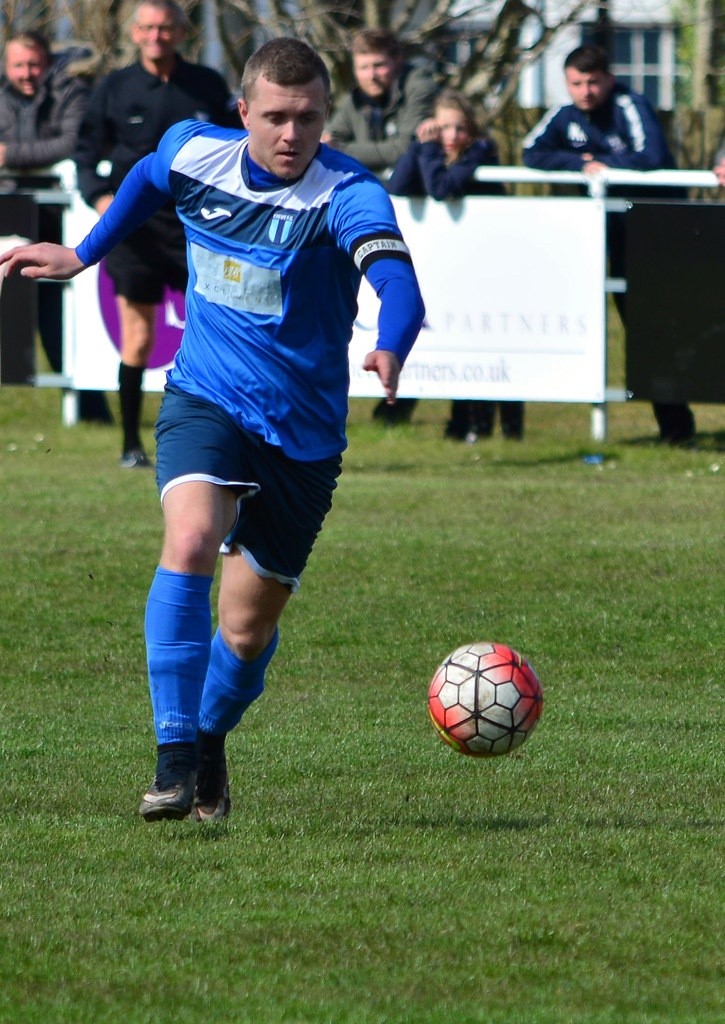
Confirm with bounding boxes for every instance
[426,640,544,759]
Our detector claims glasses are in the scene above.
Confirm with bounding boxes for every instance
[132,20,176,36]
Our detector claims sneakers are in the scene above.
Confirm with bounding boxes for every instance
[194,726,231,822]
[138,744,196,820]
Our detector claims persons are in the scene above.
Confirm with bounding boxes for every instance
[319,32,428,428]
[0,37,425,826]
[522,44,698,443]
[73,1,244,469]
[0,26,98,383]
[388,93,518,442]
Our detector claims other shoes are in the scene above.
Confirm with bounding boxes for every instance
[373,398,418,424]
[499,402,524,441]
[444,401,498,442]
[651,403,696,450]
[119,444,151,468]
